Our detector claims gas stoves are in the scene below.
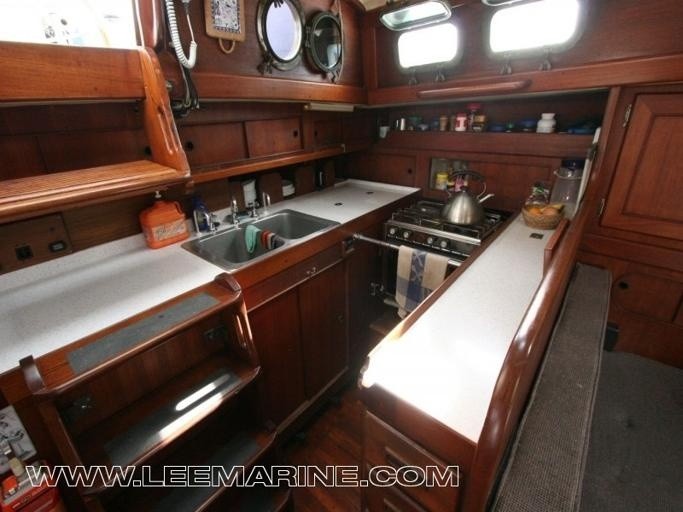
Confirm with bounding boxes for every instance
[383,199,504,262]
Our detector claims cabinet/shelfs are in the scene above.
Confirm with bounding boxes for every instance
[597,91,683,241]
[23,272,293,512]
[575,232,683,371]
[245,244,352,436]
[51,115,551,207]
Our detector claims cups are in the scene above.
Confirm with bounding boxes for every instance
[379,126,390,138]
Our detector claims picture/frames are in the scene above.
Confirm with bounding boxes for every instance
[203,0,246,56]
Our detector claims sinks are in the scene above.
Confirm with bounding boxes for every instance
[181,227,285,270]
[248,208,343,247]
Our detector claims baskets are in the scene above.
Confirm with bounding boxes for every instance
[522,204,566,230]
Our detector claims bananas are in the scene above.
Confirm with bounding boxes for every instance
[540,204,563,213]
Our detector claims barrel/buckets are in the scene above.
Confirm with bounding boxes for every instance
[142,191,188,248]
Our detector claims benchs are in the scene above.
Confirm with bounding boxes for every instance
[490,260,683,512]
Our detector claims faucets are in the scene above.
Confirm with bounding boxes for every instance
[192,206,211,233]
[262,191,271,212]
[230,197,239,223]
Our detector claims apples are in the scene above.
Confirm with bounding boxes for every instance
[530,208,540,216]
[542,207,558,216]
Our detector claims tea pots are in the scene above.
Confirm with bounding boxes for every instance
[440,170,495,227]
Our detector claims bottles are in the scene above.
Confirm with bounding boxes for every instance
[432,103,488,132]
[525,182,549,204]
[535,112,557,134]
